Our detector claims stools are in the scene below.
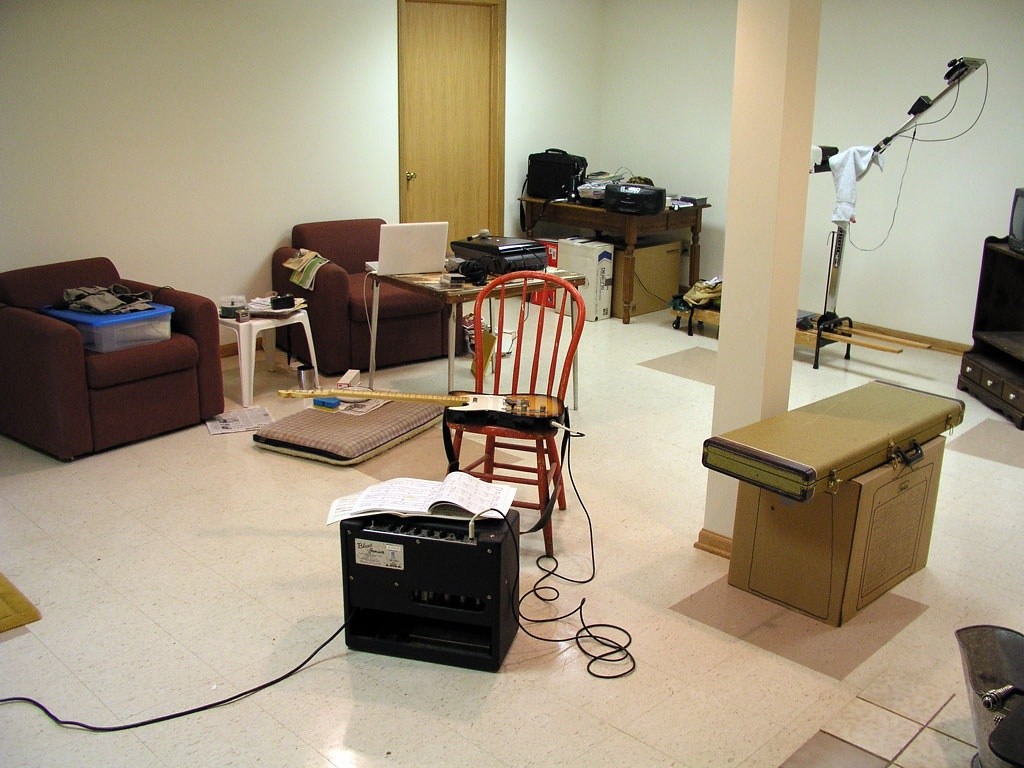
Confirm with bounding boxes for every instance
[217,307,319,407]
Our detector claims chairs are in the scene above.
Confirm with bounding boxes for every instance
[446,273,585,558]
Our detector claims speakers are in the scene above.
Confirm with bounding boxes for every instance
[339,509,520,673]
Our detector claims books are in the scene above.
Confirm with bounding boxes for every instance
[325,469,518,530]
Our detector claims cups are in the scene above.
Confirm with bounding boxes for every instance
[297,365,315,391]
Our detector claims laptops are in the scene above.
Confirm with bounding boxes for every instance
[365,222,449,276]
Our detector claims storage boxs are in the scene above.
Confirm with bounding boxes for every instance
[585,233,682,320]
[727,433,946,628]
[37,299,174,353]
[529,236,615,323]
[680,195,707,206]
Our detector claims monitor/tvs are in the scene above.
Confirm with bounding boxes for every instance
[1009,188,1024,254]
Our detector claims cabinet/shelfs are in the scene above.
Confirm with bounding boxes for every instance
[956,238,1023,431]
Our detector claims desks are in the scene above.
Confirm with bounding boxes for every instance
[518,195,712,324]
[367,269,586,411]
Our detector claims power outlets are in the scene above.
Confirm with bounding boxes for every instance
[681,239,690,256]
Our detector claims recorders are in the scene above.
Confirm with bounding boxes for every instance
[605,182,666,216]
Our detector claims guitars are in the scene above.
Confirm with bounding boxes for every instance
[275,390,566,432]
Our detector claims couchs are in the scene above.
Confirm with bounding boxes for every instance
[1,257,224,465]
[272,217,464,376]
